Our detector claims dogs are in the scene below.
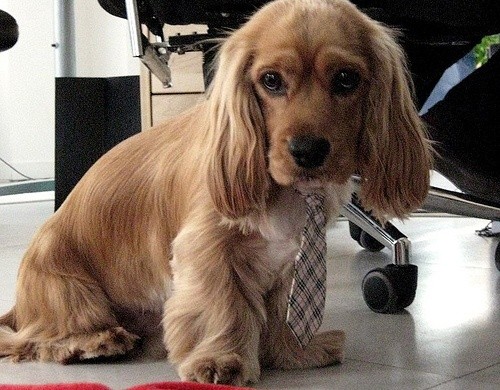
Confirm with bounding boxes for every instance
[0,0,442,387]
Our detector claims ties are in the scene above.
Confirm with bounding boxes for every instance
[286,189,329,351]
